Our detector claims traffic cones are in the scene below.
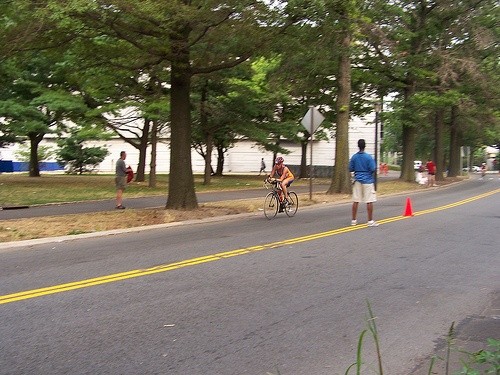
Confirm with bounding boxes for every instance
[403,197,414,217]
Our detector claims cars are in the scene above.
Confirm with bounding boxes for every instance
[463,166,482,173]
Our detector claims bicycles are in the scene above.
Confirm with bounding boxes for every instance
[264,180,298,221]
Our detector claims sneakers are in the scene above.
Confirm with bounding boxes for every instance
[351,219,358,226]
[288,202,294,206]
[367,221,379,227]
[429,183,431,187]
[433,184,438,187]
[116,205,125,209]
[278,206,284,213]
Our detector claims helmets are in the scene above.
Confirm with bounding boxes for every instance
[275,157,284,164]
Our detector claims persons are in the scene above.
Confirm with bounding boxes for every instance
[419,160,437,187]
[115,151,133,209]
[481,162,487,177]
[265,157,294,213]
[258,158,267,176]
[349,139,379,227]
[380,161,389,177]
